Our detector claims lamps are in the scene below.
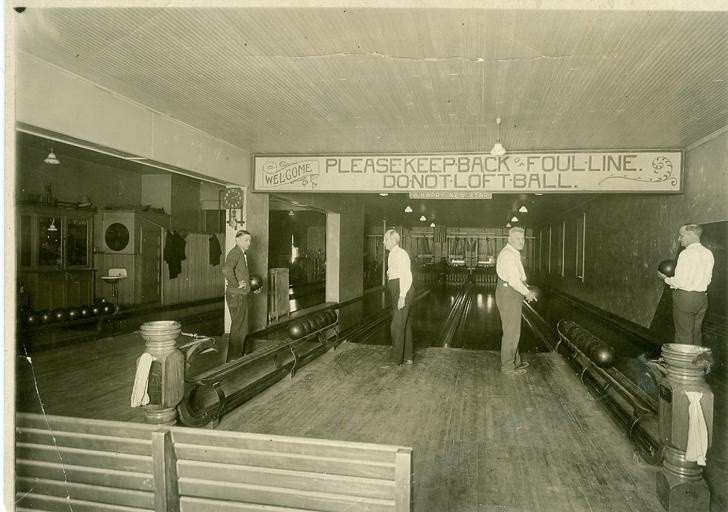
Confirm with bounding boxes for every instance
[490,117,506,156]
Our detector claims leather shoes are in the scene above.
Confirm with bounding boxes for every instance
[382,360,413,368]
[518,361,529,368]
[502,368,527,375]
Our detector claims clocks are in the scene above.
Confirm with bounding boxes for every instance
[223,188,242,229]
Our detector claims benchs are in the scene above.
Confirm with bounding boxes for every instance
[15,414,414,512]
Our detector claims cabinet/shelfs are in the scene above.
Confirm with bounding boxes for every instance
[16,204,98,312]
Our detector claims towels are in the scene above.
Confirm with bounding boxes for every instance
[683,391,708,468]
[130,353,155,408]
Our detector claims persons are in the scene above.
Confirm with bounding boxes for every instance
[379,228,415,367]
[223,230,261,360]
[495,228,537,377]
[657,224,713,346]
